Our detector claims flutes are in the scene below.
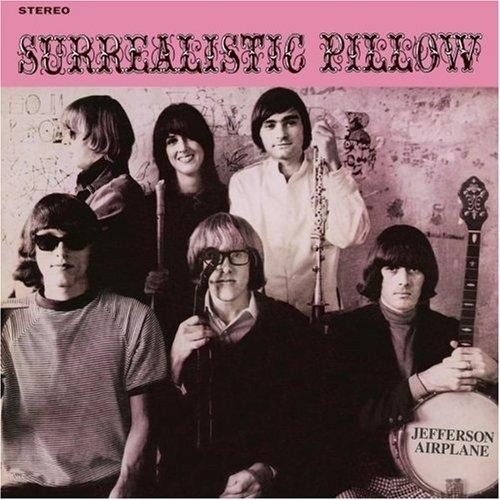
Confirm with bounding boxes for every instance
[152,178,169,329]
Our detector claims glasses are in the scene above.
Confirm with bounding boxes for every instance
[31,231,89,252]
[198,248,254,268]
[69,130,83,140]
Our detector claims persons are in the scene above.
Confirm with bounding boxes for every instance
[1,192,172,497]
[225,87,372,316]
[109,102,231,354]
[312,221,497,499]
[166,209,309,497]
[62,91,144,287]
